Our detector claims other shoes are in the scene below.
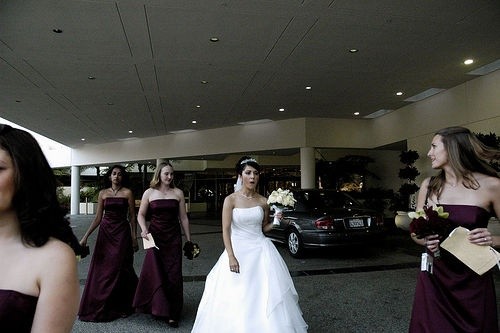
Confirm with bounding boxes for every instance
[164,318,179,327]
[121,313,128,318]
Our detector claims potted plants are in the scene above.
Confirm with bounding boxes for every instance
[389,149,422,232]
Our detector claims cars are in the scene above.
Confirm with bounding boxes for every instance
[264,188,386,259]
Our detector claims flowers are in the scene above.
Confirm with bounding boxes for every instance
[266,187,298,228]
[409,204,453,274]
[183,241,201,260]
[74,245,90,261]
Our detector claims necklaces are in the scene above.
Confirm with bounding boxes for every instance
[111,186,123,195]
[237,189,258,199]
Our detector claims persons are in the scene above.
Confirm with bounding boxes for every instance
[0,124,81,333]
[75,165,139,323]
[136,163,192,327]
[190,157,311,333]
[408,125,500,333]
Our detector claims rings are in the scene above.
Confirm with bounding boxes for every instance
[484,237,487,241]
